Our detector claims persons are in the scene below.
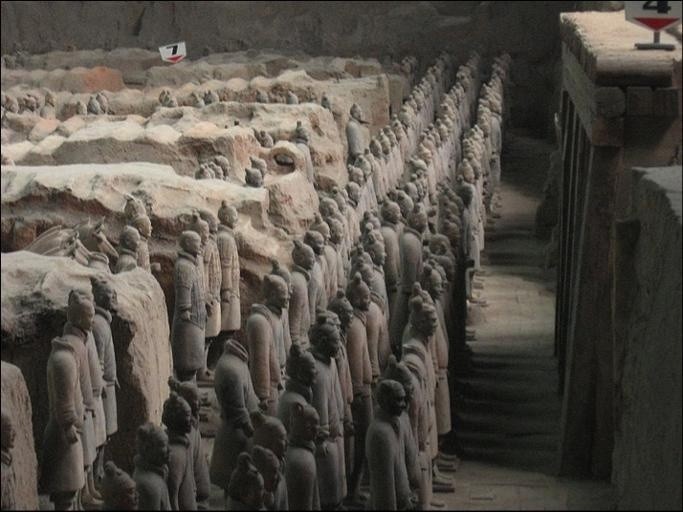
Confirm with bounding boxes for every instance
[1,43,521,511]
